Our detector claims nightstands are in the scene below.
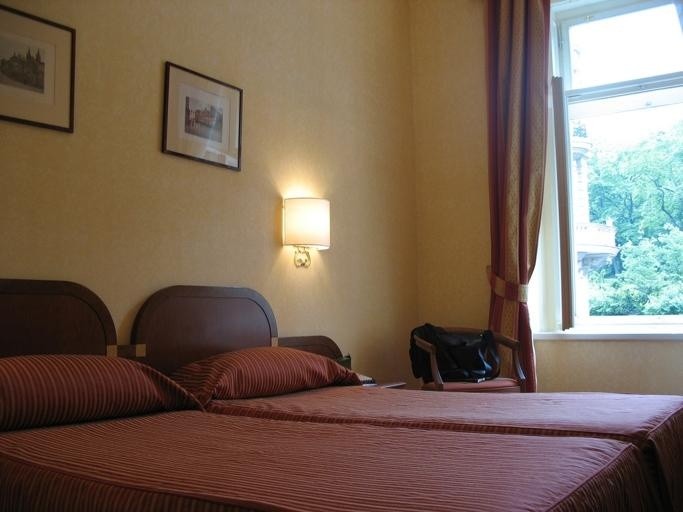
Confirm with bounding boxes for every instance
[364,381,407,390]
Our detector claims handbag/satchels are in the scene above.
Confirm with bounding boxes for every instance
[410,324,500,383]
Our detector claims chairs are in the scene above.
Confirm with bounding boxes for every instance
[413,326,527,393]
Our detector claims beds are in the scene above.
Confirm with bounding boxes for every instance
[0,278,657,512]
[129,285,683,512]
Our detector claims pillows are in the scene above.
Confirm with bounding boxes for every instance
[171,346,362,409]
[0,353,205,431]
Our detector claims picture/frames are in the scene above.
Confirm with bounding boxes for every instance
[162,61,243,172]
[0,4,76,133]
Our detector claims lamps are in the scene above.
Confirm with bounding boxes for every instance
[282,196,331,268]
